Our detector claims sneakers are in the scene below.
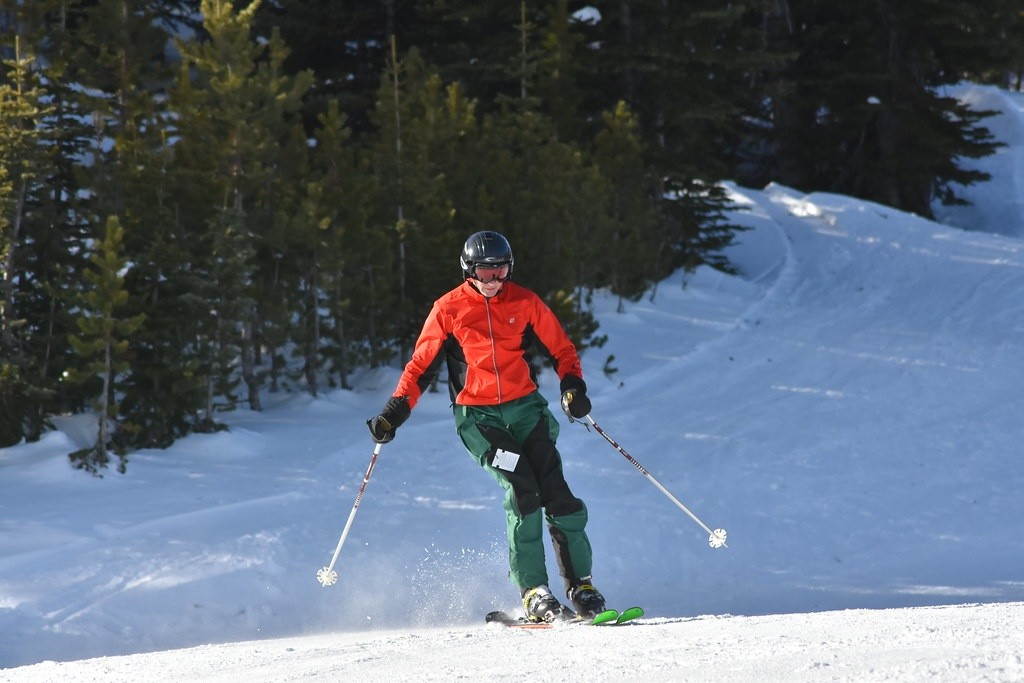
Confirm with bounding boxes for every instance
[564,574,606,613]
[520,583,561,620]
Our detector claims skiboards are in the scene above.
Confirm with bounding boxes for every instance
[485,605,643,630]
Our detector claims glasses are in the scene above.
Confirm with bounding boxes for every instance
[470,263,511,283]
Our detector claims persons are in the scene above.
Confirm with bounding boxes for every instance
[366,231,606,622]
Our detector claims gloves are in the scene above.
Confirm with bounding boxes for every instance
[560,374,592,418]
[366,395,411,443]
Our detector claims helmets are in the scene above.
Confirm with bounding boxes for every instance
[460,231,514,281]
